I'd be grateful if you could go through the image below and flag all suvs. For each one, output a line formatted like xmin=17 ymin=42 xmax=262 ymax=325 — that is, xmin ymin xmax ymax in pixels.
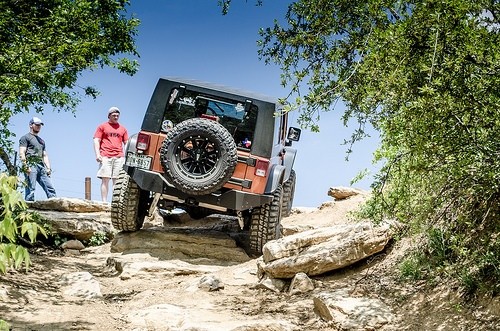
xmin=110 ymin=78 xmax=301 ymax=254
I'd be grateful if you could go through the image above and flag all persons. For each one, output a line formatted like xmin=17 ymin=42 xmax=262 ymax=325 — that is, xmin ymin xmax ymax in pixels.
xmin=94 ymin=106 xmax=128 ymax=203
xmin=18 ymin=117 xmax=58 ymax=202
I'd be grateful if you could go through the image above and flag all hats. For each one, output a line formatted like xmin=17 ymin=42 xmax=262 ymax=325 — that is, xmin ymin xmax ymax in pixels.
xmin=29 ymin=117 xmax=44 ymax=126
xmin=108 ymin=107 xmax=120 ymax=119
